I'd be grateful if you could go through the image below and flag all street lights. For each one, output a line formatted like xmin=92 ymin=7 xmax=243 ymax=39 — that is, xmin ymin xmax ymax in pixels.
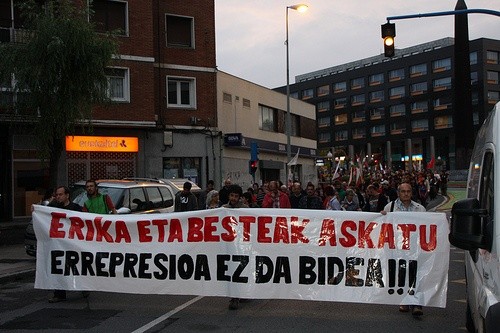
xmin=281 ymin=3 xmax=311 ymax=186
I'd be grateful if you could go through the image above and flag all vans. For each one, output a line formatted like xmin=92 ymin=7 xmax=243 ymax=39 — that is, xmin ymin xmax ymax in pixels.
xmin=449 ymin=100 xmax=500 ymax=333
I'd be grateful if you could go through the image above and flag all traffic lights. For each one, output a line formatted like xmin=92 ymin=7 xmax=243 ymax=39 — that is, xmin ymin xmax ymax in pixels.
xmin=248 ymin=160 xmax=257 ymax=175
xmin=381 ymin=23 xmax=396 ymax=58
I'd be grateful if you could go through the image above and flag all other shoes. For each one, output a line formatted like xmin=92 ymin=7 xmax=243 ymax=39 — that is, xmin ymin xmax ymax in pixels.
xmin=410 ymin=306 xmax=423 ymax=315
xmin=228 ymin=299 xmax=240 ymax=310
xmin=47 ymin=295 xmax=66 ymax=303
xmin=399 ymin=305 xmax=409 ymax=312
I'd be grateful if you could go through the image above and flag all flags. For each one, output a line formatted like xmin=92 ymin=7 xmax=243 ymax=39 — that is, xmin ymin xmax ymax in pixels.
xmin=287 ymin=151 xmax=298 ymax=165
xmin=332 ymin=158 xmax=385 ymax=186
xmin=427 ymin=157 xmax=435 ymax=170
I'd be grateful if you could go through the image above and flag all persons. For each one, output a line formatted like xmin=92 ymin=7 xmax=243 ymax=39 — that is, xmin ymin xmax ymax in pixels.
xmin=206 ymin=190 xmax=219 ymax=209
xmin=262 ymin=180 xmax=323 ymax=210
xmin=83 ymin=180 xmax=117 ymax=214
xmin=30 ymin=186 xmax=90 ymax=303
xmin=222 ymin=184 xmax=251 ymax=310
xmin=389 ymin=160 xmax=448 ymax=208
xmin=320 ymin=182 xmax=389 ymax=213
xmin=241 ymin=183 xmax=265 ymax=208
xmin=197 ymin=180 xmax=215 ymax=210
xmin=219 ymin=178 xmax=232 ymax=204
xmin=319 ymin=161 xmax=390 ymax=193
xmin=175 ymin=181 xmax=198 ymax=213
xmin=380 ymin=182 xmax=426 ymax=316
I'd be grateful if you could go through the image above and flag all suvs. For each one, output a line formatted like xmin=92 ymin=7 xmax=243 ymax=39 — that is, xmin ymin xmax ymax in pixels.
xmin=25 ymin=176 xmax=177 ymax=259
